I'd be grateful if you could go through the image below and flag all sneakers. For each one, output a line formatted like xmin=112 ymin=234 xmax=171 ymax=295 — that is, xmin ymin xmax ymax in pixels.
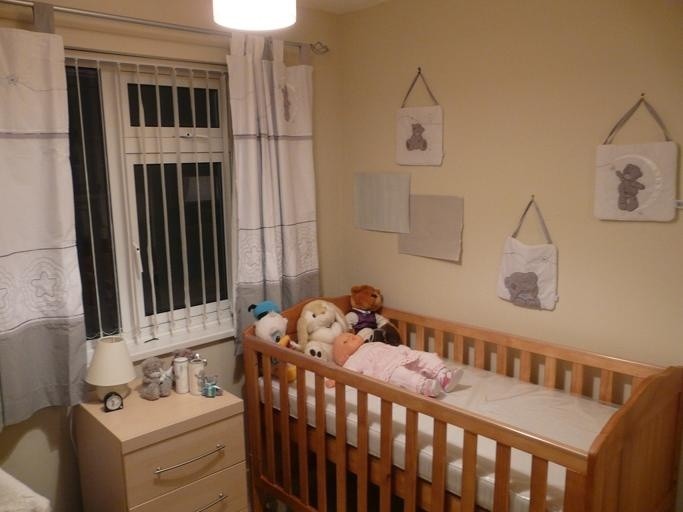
xmin=439 ymin=369 xmax=464 ymax=392
xmin=424 ymin=379 xmax=440 ymax=398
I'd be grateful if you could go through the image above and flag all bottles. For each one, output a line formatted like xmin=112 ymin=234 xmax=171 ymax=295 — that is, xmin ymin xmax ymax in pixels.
xmin=175 ymin=357 xmax=204 ymax=396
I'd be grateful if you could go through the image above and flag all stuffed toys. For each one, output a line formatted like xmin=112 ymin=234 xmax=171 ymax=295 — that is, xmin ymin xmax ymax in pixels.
xmin=141 ymin=357 xmax=174 ymax=400
xmin=246 ymin=301 xmax=299 ymax=383
xmin=344 ymin=284 xmax=399 ymax=346
xmin=296 ymin=299 xmax=350 ymax=362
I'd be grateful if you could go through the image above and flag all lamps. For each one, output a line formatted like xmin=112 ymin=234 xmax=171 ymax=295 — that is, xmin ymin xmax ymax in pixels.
xmin=83 ymin=335 xmax=136 ymax=401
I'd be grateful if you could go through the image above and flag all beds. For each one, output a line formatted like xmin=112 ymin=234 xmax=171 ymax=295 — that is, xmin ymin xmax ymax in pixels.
xmin=248 ymin=294 xmax=681 ymax=511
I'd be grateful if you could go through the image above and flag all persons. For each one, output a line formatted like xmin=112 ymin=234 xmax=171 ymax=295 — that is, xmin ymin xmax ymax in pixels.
xmin=324 ymin=331 xmax=465 ymax=397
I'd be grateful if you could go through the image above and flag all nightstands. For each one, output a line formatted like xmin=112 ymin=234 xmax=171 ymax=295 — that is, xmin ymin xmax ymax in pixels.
xmin=71 ymin=386 xmax=249 ymax=512
